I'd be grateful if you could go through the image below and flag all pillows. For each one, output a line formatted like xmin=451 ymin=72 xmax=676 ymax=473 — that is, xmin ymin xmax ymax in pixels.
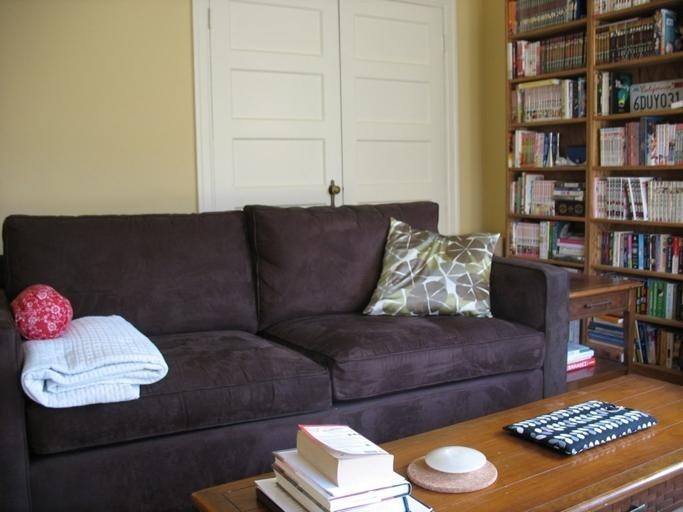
xmin=363 ymin=215 xmax=500 ymax=318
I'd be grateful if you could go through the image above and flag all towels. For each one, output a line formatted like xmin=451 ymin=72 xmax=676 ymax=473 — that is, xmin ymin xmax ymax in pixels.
xmin=11 ymin=284 xmax=168 ymax=409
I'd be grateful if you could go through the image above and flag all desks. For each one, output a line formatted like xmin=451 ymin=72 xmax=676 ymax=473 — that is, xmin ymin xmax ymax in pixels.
xmin=191 ymin=370 xmax=683 ymax=512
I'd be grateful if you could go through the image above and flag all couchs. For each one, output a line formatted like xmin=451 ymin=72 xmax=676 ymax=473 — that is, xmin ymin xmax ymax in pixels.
xmin=0 ymin=202 xmax=569 ymax=512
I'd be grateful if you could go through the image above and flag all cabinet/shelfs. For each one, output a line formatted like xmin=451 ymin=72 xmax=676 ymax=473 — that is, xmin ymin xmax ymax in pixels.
xmin=503 ymin=0 xmax=683 ymax=382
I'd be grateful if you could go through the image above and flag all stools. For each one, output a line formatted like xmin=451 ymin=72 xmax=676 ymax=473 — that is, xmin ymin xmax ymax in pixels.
xmin=567 ymin=276 xmax=644 ymax=377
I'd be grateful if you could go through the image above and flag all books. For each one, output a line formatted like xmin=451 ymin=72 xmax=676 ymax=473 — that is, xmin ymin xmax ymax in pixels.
xmin=503 ymin=1 xmax=586 ymax=124
xmin=592 ymin=117 xmax=682 ymax=224
xmin=508 ymin=128 xmax=586 ymax=272
xmin=593 ymin=1 xmax=683 ymax=115
xmin=567 ymin=230 xmax=682 ymax=372
xmin=251 ymin=422 xmax=432 ymax=512
